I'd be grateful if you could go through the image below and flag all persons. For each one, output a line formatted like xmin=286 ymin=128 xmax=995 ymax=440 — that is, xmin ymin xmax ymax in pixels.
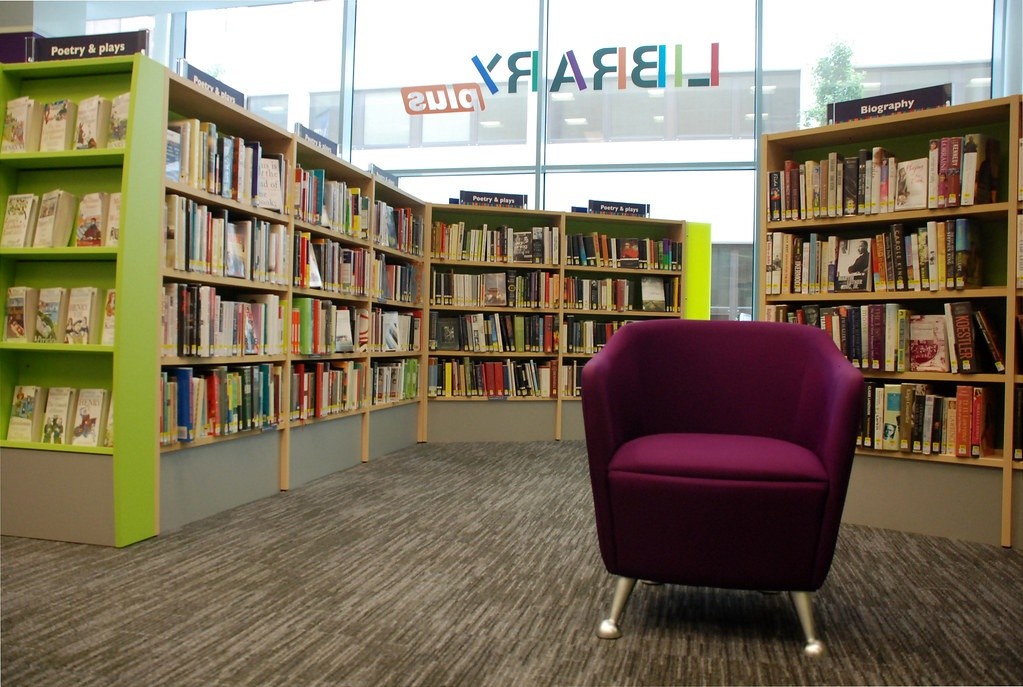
xmin=949 ymin=401 xmax=956 ymax=410
xmin=805 ymin=307 xmax=818 ymax=327
xmin=848 ymin=240 xmax=869 ymax=289
xmin=883 ymin=423 xmax=895 ymax=440
xmin=965 ymin=136 xmax=977 ymax=152
xmin=839 ymin=241 xmax=847 ymax=254
xmin=930 ymin=140 xmax=938 ymax=152
xmin=977 ymin=139 xmax=1001 ymax=203
xmin=882 ymin=159 xmax=888 ymax=167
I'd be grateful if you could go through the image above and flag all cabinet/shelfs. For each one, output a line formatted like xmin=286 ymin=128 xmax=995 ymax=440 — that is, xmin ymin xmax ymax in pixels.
xmin=757 ymin=95 xmax=1023 ymax=548
xmin=1 ymin=51 xmax=713 ymax=548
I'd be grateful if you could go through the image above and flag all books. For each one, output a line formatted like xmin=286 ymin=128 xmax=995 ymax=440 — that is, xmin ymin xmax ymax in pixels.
xmin=0 ymin=93 xmax=684 ymax=447
xmin=765 ymin=135 xmax=1022 ymax=461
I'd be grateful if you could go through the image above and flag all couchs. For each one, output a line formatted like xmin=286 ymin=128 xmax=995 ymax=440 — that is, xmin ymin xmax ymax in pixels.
xmin=581 ymin=321 xmax=865 ymax=661
